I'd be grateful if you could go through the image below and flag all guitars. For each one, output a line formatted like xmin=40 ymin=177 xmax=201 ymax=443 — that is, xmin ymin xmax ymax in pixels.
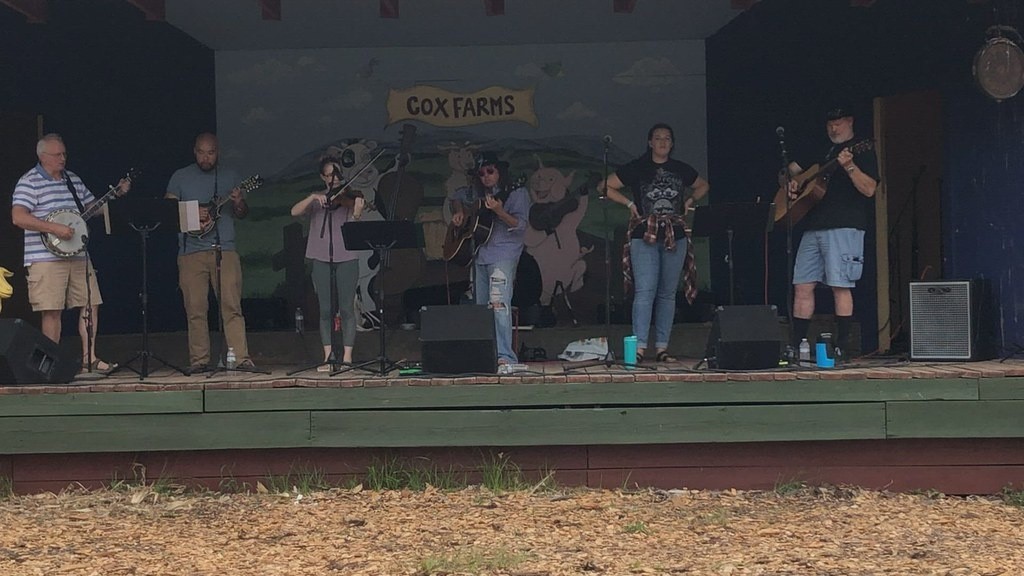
xmin=39 ymin=166 xmax=144 ymax=257
xmin=771 ymin=137 xmax=878 ymax=233
xmin=185 ymin=172 xmax=265 ymax=236
xmin=443 ymin=172 xmax=527 ymax=269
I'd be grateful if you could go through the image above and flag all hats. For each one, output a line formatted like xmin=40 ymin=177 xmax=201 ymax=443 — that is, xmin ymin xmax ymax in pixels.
xmin=468 ymin=150 xmax=510 ymax=176
xmin=824 ymin=103 xmax=852 ymax=121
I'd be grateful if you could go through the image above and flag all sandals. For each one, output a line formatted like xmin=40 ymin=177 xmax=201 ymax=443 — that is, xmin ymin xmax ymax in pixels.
xmin=657 ymin=350 xmax=676 ymax=362
xmin=637 ymin=353 xmax=645 ymax=363
xmin=80 ymin=357 xmax=119 ymax=373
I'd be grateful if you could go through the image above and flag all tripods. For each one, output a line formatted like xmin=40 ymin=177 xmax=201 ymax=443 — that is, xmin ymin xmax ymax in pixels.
xmin=106 ymin=223 xmax=189 ymax=378
xmin=195 ymin=200 xmax=272 ymax=378
xmin=330 ymin=219 xmax=425 ymax=377
xmin=565 ymin=152 xmax=655 ymax=371
xmin=878 ymin=173 xmax=925 ymax=356
xmin=284 ymin=205 xmax=380 ymax=375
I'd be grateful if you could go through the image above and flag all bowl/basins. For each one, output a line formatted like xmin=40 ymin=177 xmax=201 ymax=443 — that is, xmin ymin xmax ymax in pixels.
xmin=402 ymin=323 xmax=415 ymax=330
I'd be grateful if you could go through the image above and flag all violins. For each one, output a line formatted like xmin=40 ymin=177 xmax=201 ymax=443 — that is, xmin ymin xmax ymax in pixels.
xmin=342 ymin=183 xmax=376 ymax=212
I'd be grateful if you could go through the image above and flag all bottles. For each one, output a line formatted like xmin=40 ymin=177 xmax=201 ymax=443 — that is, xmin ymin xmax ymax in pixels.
xmin=799 ymin=338 xmax=810 ymax=368
xmin=227 ymin=347 xmax=236 ymax=377
xmin=295 ymin=308 xmax=305 ymax=333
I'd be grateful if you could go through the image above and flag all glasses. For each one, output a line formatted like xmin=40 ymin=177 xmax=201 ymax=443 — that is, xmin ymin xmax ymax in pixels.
xmin=476 ymin=169 xmax=496 ymax=178
xmin=43 ymin=152 xmax=66 ymax=159
xmin=323 ymin=173 xmax=333 ymax=178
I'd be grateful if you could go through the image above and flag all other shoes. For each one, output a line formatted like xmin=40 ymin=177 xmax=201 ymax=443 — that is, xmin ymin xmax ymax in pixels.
xmin=498 ymin=357 xmax=508 ymax=365
xmin=238 ymin=363 xmax=252 ymax=369
xmin=830 ymin=346 xmax=846 ymax=362
xmin=316 ymin=362 xmax=337 ymax=372
xmin=340 ymin=363 xmax=351 ymax=371
xmin=191 ymin=366 xmax=207 ymax=373
xmin=783 ymin=345 xmax=800 ymax=361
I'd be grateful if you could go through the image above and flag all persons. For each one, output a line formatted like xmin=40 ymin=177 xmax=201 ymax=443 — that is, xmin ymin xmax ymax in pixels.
xmin=290 ymin=156 xmax=365 ymax=372
xmin=452 ymin=151 xmax=531 ymax=366
xmin=12 ymin=133 xmax=130 ymax=375
xmin=596 ymin=124 xmax=709 ymax=363
xmin=159 ymin=133 xmax=257 ymax=372
xmin=785 ymin=105 xmax=882 ymax=365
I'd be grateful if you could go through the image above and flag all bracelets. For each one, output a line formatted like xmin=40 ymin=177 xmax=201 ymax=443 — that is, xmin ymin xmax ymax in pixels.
xmin=690 ymin=195 xmax=698 ymax=204
xmin=627 ymin=201 xmax=634 ymax=209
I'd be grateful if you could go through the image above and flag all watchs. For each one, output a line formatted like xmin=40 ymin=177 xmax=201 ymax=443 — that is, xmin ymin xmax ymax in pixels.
xmin=847 ymin=164 xmax=857 ymax=175
xmin=352 ymin=212 xmax=361 ymax=219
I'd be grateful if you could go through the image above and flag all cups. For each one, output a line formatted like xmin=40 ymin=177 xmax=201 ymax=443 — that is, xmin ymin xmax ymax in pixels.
xmin=624 ymin=336 xmax=638 ymax=369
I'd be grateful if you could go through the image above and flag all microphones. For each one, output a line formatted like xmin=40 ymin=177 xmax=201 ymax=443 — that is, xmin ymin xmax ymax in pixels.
xmin=601 ymin=135 xmax=613 ymax=144
xmin=333 ymin=165 xmax=346 ymax=185
xmin=775 ymin=126 xmax=785 ymax=143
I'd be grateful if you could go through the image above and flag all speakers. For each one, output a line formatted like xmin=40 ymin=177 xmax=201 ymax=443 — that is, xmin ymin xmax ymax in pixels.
xmin=419 ymin=304 xmax=498 ymax=375
xmin=0 ymin=319 xmax=58 ymax=385
xmin=705 ymin=304 xmax=781 ymax=370
xmin=909 ymin=280 xmax=973 ymax=360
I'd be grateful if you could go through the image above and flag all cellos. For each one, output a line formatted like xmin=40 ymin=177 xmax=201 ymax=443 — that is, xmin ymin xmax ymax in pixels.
xmin=370 ymin=123 xmax=425 ymax=296
